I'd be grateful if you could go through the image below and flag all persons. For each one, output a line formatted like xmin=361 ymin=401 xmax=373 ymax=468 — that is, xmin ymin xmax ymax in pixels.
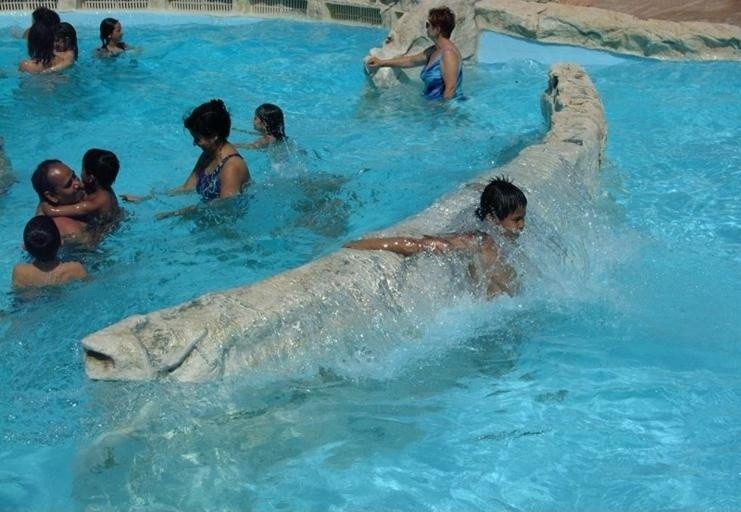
xmin=233 ymin=104 xmax=288 ymax=150
xmin=0 ymin=138 xmax=127 ymax=289
xmin=18 ymin=7 xmax=78 ymax=75
xmin=96 ymin=18 xmax=144 ymax=57
xmin=341 ymin=174 xmax=528 ymax=301
xmin=367 ymin=7 xmax=467 ymax=102
xmin=120 ymin=99 xmax=250 ymax=222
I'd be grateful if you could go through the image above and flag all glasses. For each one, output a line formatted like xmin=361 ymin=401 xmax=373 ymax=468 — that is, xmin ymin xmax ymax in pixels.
xmin=426 ymin=22 xmax=431 ymax=28
